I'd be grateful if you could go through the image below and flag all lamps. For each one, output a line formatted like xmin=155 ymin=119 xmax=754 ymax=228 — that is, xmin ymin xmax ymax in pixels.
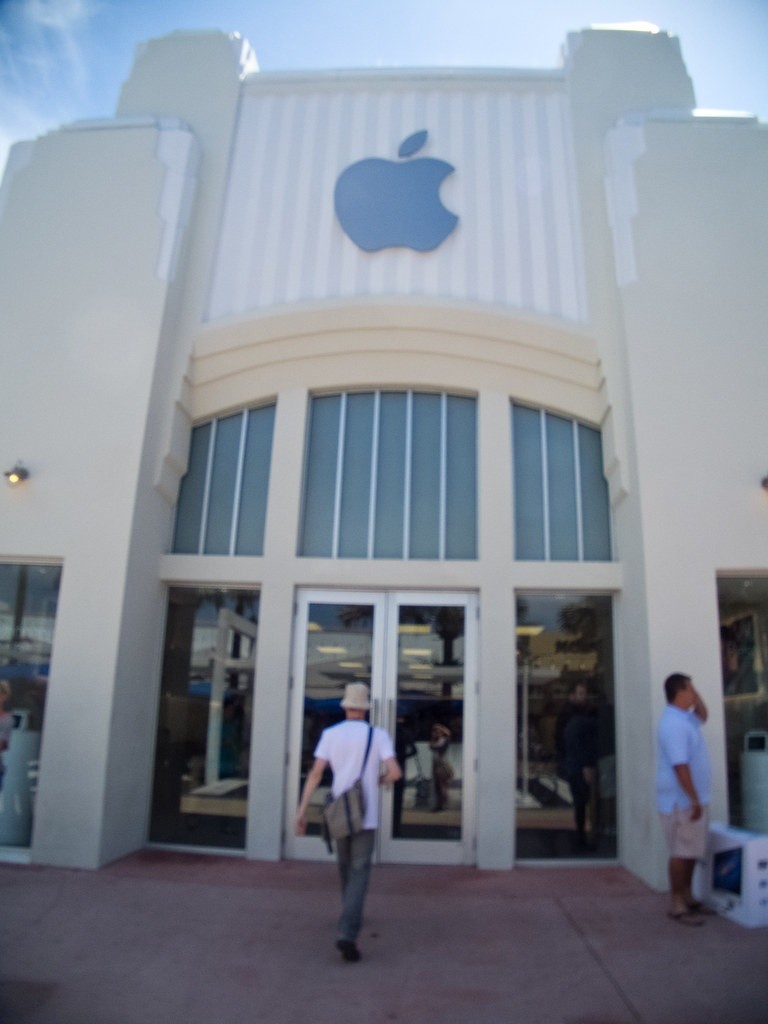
xmin=3 ymin=464 xmax=28 ymax=484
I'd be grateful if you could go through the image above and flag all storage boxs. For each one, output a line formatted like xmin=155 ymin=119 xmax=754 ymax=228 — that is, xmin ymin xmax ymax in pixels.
xmin=692 ymin=821 xmax=768 ymax=929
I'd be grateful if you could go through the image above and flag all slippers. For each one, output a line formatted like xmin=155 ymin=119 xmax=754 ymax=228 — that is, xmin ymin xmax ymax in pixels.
xmin=667 ymin=909 xmax=704 ymax=926
xmin=689 ymin=901 xmax=715 ymax=915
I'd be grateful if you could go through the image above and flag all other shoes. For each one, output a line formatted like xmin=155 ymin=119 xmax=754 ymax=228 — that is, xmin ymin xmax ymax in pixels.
xmin=336 ymin=940 xmax=361 ymax=962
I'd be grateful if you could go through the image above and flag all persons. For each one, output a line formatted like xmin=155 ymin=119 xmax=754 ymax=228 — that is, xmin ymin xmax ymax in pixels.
xmin=296 ymin=682 xmax=403 ymax=964
xmin=654 ymin=674 xmax=716 ymax=925
xmin=555 ymin=684 xmax=606 ymax=840
xmin=0 ymin=679 xmax=15 ymax=793
xmin=431 ymin=724 xmax=454 ymax=813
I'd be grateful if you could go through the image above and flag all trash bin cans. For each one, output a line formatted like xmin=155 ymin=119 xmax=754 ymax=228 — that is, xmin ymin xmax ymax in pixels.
xmin=0 ymin=709 xmax=39 ymax=845
xmin=741 ymin=729 xmax=768 ymax=834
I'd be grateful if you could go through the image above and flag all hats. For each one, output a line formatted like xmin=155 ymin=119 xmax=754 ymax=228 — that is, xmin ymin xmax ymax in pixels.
xmin=340 ymin=683 xmax=371 ymax=710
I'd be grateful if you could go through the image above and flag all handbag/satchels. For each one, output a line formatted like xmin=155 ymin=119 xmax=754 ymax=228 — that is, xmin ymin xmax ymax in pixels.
xmin=323 ymin=782 xmax=367 ymax=840
